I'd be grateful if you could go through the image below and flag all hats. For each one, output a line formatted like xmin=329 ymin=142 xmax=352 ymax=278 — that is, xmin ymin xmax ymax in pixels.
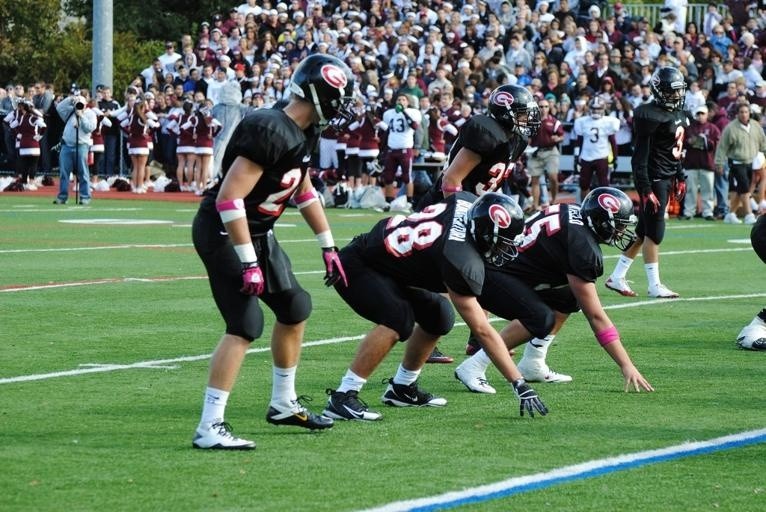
xmin=693 ymin=104 xmax=708 ymax=115
xmin=96 ymin=84 xmax=105 ymax=91
xmin=754 ymin=79 xmax=766 ymax=87
xmin=201 ymin=3 xmax=684 ymax=106
xmin=165 ymin=41 xmax=174 ymax=48
xmin=70 ymin=82 xmax=81 ymax=92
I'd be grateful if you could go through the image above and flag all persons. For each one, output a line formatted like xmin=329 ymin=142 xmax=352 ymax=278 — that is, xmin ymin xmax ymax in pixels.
xmin=736 ymin=214 xmax=766 ymax=350
xmin=0 ymin=81 xmax=222 ymax=195
xmin=324 ymin=192 xmax=548 ymax=420
xmin=454 ymin=188 xmax=654 ymax=394
xmin=456 ymin=0 xmax=766 ymax=191
xmin=528 ymin=99 xmax=563 ymax=214
xmin=136 ymin=0 xmax=455 ymax=209
xmin=605 ymin=69 xmax=687 ymax=297
xmin=54 ymin=94 xmax=97 ymax=205
xmin=574 ymin=96 xmax=619 ymax=203
xmin=684 ymin=100 xmax=766 ymax=225
xmin=417 ymin=85 xmax=540 ymax=361
xmin=382 ymin=95 xmax=421 ymax=211
xmin=194 ymin=55 xmax=355 ymax=456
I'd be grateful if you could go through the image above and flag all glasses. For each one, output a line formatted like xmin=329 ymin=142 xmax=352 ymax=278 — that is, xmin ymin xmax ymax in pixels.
xmin=538 ymin=104 xmax=549 ymax=109
xmin=5 ymin=85 xmax=43 ymax=92
xmin=661 ymin=87 xmax=682 ymax=95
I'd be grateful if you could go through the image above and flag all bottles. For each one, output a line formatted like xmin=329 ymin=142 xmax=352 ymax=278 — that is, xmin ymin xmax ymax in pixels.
xmin=395 ymin=103 xmax=402 ymax=114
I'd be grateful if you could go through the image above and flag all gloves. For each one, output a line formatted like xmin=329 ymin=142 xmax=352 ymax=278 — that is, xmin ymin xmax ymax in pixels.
xmin=673 ymin=177 xmax=687 ymax=203
xmin=238 ymin=260 xmax=266 ymax=297
xmin=319 ymin=245 xmax=350 ymax=289
xmin=511 ymin=377 xmax=550 ymax=419
xmin=641 ymin=190 xmax=662 ymax=216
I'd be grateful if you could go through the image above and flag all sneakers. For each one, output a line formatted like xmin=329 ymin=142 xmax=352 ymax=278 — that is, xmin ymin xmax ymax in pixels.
xmin=646 ymin=282 xmax=681 ymax=298
xmin=603 ymin=275 xmax=639 ymax=298
xmin=131 ymin=179 xmax=155 ymax=194
xmin=722 ymin=212 xmax=742 ymax=225
xmin=23 ymin=179 xmax=45 ymax=191
xmin=744 ymin=212 xmax=757 ymax=225
xmin=53 ymin=199 xmax=68 ymax=205
xmin=379 ymin=376 xmax=449 ymax=409
xmin=463 ymin=341 xmax=517 ymax=359
xmin=262 ymin=395 xmax=335 ymax=431
xmin=425 ymin=344 xmax=454 ymax=364
xmin=452 ymin=366 xmax=498 ymax=397
xmin=513 ymin=363 xmax=574 ymax=385
xmin=733 ymin=322 xmax=766 ymax=353
xmin=179 ymin=180 xmax=209 ymax=196
xmin=321 ymin=388 xmax=384 ymax=422
xmin=78 ymin=198 xmax=92 ymax=206
xmin=677 ymin=213 xmax=695 ymax=223
xmin=191 ymin=420 xmax=258 ymax=452
xmin=704 ymin=214 xmax=718 ymax=222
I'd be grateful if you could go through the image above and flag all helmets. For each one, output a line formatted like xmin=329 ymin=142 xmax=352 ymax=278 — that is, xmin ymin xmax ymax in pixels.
xmin=487 ymin=83 xmax=543 ymax=138
xmin=649 ymin=66 xmax=688 ymax=111
xmin=287 ymin=52 xmax=357 ymax=131
xmin=587 ymin=96 xmax=606 ymax=120
xmin=577 ymin=185 xmax=639 ymax=254
xmin=464 ymin=190 xmax=526 ymax=267
